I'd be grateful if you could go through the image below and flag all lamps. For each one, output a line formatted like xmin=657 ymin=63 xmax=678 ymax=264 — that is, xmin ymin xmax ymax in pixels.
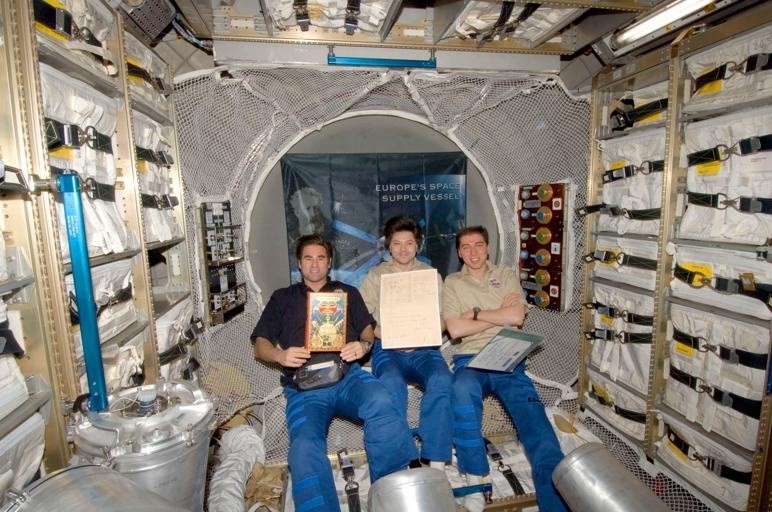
xmin=609 ymin=0 xmax=718 ymax=52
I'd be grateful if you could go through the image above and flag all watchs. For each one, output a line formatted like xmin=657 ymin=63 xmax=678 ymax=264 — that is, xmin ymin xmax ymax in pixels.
xmin=471 ymin=306 xmax=482 ymax=321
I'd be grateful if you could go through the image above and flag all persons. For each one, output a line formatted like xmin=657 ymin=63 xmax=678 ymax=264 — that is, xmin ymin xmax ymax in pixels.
xmin=249 ymin=232 xmax=416 ymax=510
xmin=286 ymin=186 xmax=359 ymax=270
xmin=356 ymin=217 xmax=454 ymax=473
xmin=443 ymin=224 xmax=571 ymax=512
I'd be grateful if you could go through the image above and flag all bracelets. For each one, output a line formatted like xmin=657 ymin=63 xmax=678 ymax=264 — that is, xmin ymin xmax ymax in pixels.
xmin=360 ymin=338 xmax=372 ymax=353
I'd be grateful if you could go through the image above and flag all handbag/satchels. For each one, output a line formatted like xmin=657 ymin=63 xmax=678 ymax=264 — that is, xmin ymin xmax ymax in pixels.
xmin=295 ymin=353 xmax=343 ymax=390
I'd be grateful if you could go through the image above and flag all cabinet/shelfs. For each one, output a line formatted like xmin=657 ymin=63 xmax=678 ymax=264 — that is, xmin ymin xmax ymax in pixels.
xmin=1 ymin=0 xmax=206 ymax=481
xmin=579 ymin=17 xmax=772 ymax=509
xmin=212 ymin=0 xmax=655 ymax=59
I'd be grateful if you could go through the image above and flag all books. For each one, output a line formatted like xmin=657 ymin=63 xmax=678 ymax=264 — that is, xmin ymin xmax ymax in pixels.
xmin=302 ymin=289 xmax=348 ymax=354
